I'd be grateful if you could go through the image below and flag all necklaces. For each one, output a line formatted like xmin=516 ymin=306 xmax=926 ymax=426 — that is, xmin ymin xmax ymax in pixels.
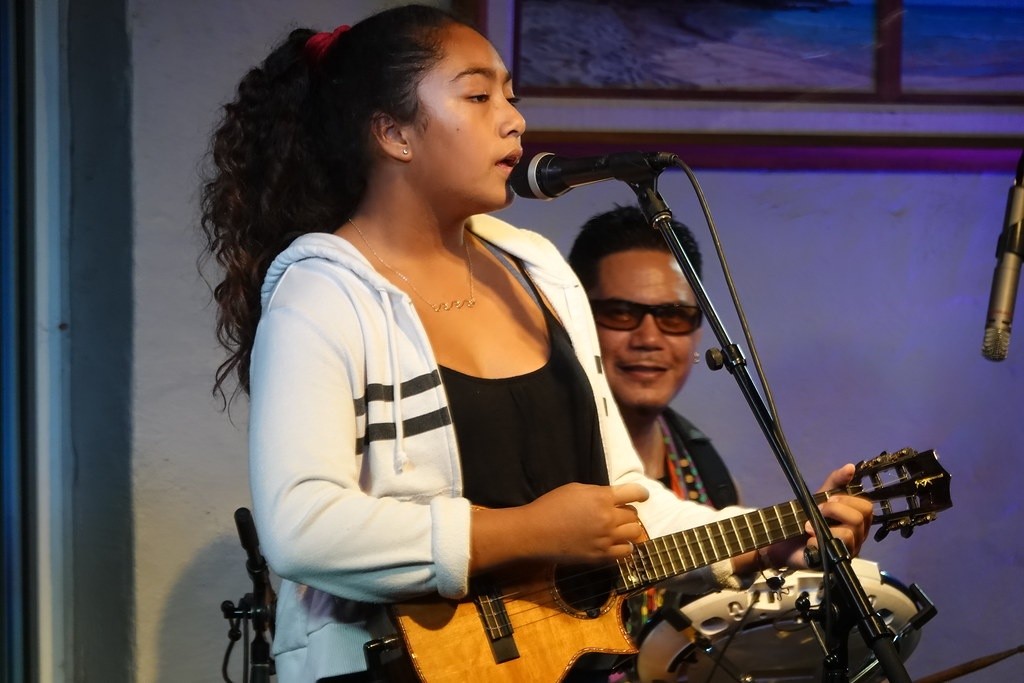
xmin=346 ymin=213 xmax=477 ymax=314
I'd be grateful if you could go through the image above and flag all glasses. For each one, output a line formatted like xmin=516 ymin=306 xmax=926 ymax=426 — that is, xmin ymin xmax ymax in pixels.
xmin=587 ymin=299 xmax=701 ymax=336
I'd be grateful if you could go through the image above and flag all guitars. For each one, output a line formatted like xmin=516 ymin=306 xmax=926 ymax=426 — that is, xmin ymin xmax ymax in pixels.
xmin=387 ymin=445 xmax=952 ymax=683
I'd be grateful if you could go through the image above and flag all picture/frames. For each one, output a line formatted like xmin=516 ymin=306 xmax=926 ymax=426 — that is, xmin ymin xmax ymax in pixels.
xmin=475 ymin=0 xmax=1024 ymax=175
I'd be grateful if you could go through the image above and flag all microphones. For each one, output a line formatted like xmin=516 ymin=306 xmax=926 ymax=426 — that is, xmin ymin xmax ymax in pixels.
xmin=510 ymin=151 xmax=674 ymax=201
xmin=982 ymin=185 xmax=1024 ymax=362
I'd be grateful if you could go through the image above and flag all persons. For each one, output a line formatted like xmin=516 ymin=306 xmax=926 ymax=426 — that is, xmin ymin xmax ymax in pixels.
xmin=565 ymin=202 xmax=741 ymax=638
xmin=199 ymin=6 xmax=873 ymax=682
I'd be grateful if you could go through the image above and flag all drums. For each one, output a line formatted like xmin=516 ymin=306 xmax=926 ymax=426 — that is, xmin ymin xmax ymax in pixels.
xmin=617 ymin=534 xmax=923 ymax=682
